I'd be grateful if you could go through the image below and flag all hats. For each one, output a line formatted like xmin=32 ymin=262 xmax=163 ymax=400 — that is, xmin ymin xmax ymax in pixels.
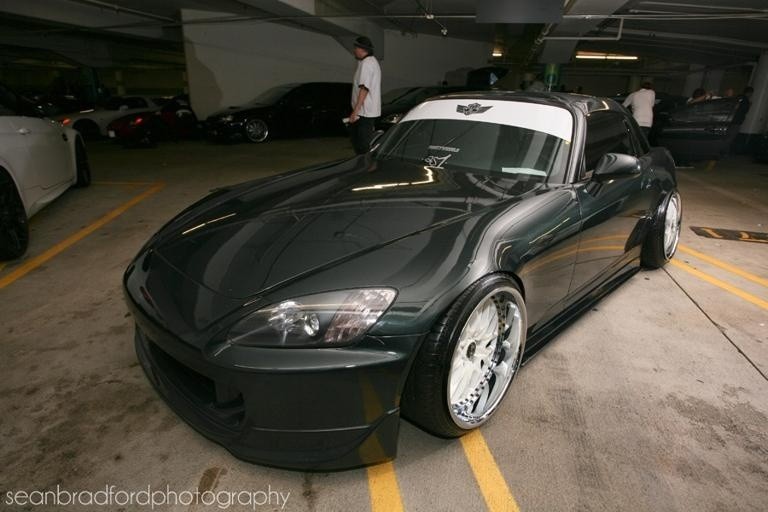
xmin=352 ymin=36 xmax=375 ymax=49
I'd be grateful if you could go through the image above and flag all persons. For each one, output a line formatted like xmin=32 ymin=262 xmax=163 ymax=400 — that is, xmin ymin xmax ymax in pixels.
xmin=347 ymin=36 xmax=384 ymax=153
xmin=491 ymin=64 xmax=751 ymax=127
xmin=623 ymin=82 xmax=654 ymax=147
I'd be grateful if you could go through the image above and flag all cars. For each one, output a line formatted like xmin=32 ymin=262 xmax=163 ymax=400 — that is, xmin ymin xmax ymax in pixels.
xmin=204 ymin=83 xmax=353 ymax=142
xmin=610 ymin=92 xmax=744 ymax=167
xmin=374 ymin=68 xmax=513 ymax=137
xmin=50 ymin=94 xmax=162 ymax=138
xmin=0 ymin=80 xmax=92 ymax=259
xmin=106 ymin=94 xmax=191 ymax=146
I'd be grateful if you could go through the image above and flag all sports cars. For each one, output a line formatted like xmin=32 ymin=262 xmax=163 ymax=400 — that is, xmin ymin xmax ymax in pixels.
xmin=122 ymin=90 xmax=683 ymax=473
